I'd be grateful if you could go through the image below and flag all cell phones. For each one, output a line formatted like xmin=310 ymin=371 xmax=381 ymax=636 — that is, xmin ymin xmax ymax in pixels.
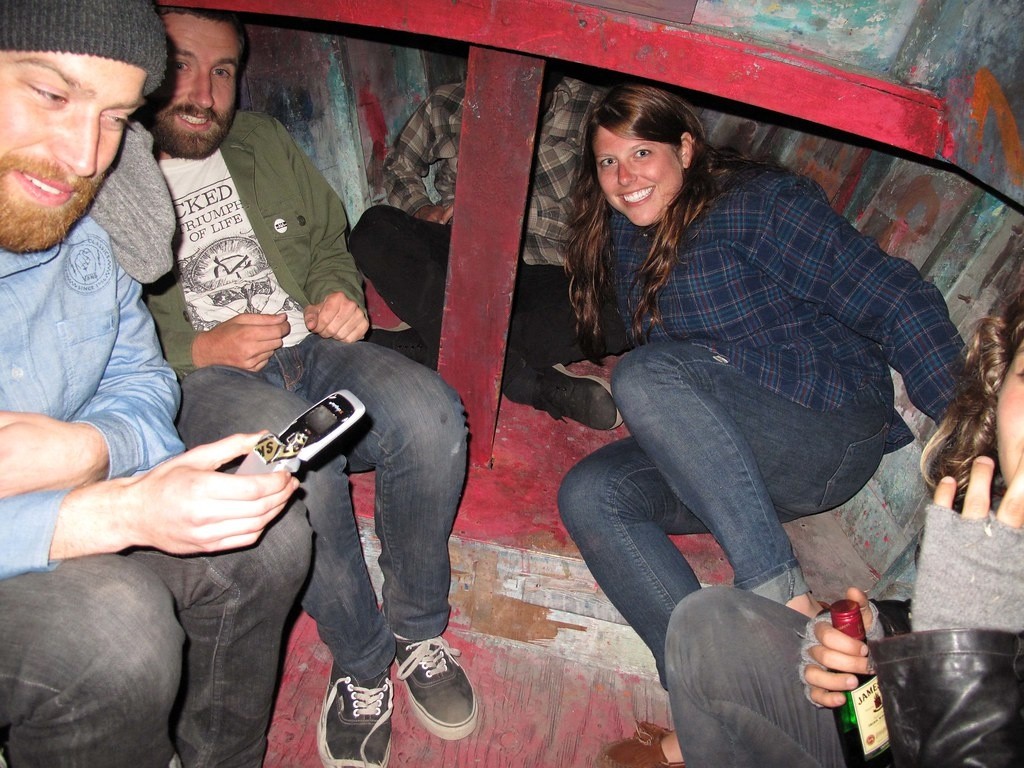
xmin=234 ymin=389 xmax=366 ymax=475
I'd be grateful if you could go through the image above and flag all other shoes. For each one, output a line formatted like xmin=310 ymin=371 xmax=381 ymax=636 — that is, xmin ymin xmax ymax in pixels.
xmin=594 ymin=720 xmax=687 ymax=768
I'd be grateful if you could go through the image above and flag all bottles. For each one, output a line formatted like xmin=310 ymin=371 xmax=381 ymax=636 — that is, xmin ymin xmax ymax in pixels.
xmin=826 ymin=599 xmax=892 ymax=768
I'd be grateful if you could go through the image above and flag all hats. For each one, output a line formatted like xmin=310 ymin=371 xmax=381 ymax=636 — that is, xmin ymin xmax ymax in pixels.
xmin=0 ymin=0 xmax=166 ymax=96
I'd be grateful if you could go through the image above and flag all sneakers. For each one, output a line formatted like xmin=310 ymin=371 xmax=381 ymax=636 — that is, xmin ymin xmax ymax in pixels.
xmin=316 ymin=660 xmax=393 ymax=768
xmin=548 ymin=363 xmax=623 ymax=430
xmin=381 ymin=605 xmax=478 ymax=740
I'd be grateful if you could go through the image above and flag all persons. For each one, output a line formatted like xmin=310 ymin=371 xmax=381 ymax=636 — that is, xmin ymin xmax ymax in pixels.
xmin=1 ymin=0 xmax=315 ymax=768
xmin=349 ymin=75 xmax=624 ymax=431
xmin=666 ymin=291 xmax=1023 ymax=767
xmin=143 ymin=10 xmax=477 ymax=768
xmin=557 ymin=86 xmax=967 ymax=767
